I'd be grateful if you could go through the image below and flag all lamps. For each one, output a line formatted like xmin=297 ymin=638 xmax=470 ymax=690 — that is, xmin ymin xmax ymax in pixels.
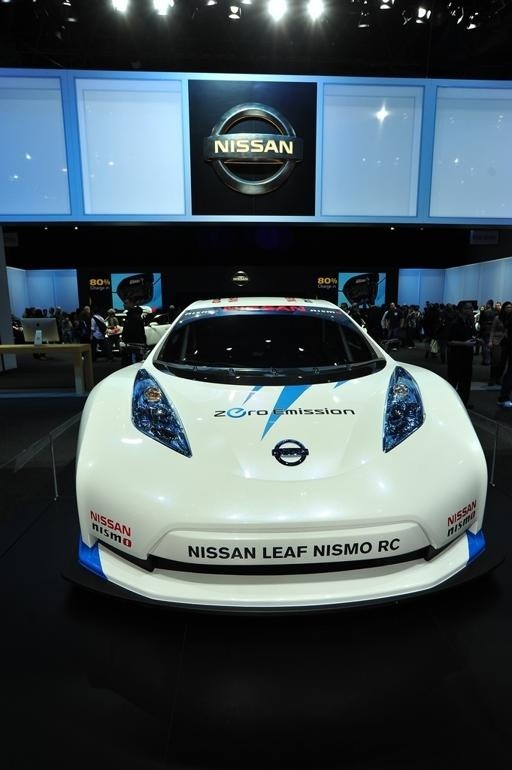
xmin=227 ymin=0 xmax=248 ymax=21
xmin=347 ymin=0 xmax=482 ymax=31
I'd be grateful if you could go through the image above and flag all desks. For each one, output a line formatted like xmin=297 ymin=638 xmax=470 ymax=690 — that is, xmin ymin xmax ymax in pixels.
xmin=0 ymin=340 xmax=94 ymax=400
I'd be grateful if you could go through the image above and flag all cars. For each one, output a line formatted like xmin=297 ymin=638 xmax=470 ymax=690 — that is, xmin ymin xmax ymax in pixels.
xmin=90 ymin=305 xmax=176 ymax=357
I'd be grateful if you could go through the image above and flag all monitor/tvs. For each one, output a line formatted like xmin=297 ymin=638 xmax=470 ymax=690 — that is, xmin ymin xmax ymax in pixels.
xmin=21 ymin=317 xmax=60 ymax=345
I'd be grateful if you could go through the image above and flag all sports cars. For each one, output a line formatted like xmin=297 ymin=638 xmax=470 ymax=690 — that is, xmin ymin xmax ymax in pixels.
xmin=70 ymin=294 xmax=490 ymax=623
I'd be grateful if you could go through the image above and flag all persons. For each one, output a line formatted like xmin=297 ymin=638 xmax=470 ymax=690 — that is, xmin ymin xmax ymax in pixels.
xmin=342 ymin=299 xmax=512 ymax=408
xmin=23 ymin=298 xmax=175 ymax=364
